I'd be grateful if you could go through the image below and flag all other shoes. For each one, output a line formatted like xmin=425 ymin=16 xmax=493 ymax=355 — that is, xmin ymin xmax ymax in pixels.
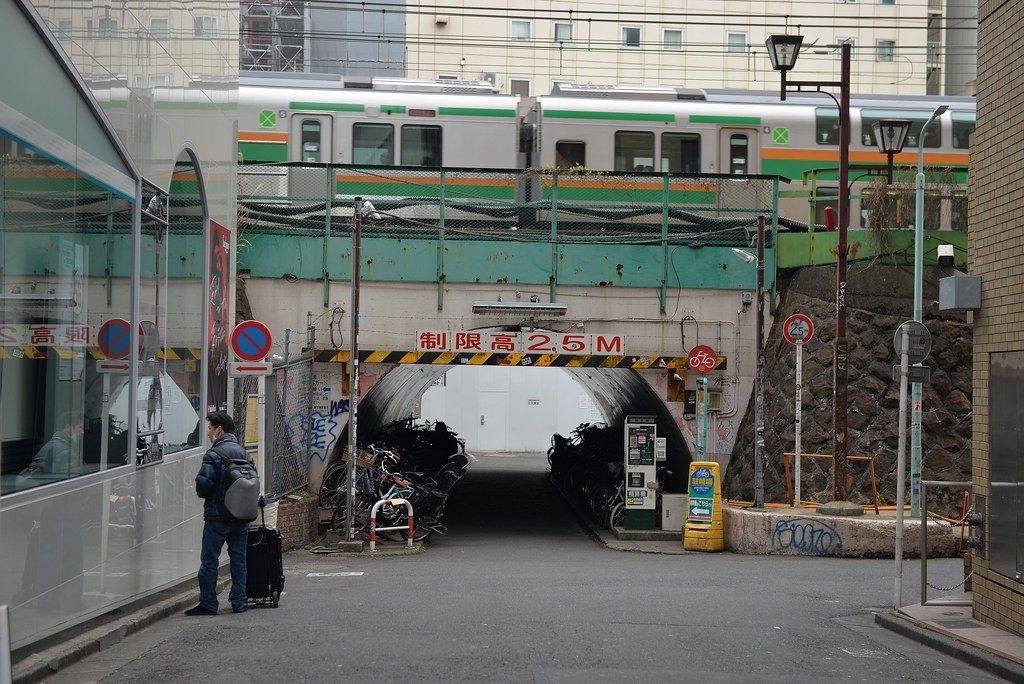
xmin=233 ymin=604 xmax=246 ymax=613
xmin=185 ymin=603 xmax=218 ymax=616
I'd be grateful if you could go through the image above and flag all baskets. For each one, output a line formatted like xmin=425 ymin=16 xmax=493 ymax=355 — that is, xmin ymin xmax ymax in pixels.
xmin=341 ymin=445 xmax=377 ymax=467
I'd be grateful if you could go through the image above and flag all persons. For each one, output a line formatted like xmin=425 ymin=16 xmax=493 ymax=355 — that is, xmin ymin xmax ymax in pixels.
xmin=185 ymin=412 xmax=255 ymax=614
xmin=28 ymin=410 xmax=91 ymax=615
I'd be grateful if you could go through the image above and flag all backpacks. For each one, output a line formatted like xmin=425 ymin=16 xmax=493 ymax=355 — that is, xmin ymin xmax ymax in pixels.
xmin=15 ymin=444 xmax=71 ymax=491
xmin=208 ymin=447 xmax=260 ymax=520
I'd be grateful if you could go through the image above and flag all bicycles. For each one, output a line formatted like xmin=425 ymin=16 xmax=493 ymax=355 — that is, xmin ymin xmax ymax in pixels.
xmin=319 ymin=411 xmax=472 ymax=543
xmin=547 ymin=421 xmax=674 ymax=532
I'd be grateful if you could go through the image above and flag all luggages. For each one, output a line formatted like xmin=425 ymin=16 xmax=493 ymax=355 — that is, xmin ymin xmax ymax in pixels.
xmin=242 ymin=500 xmax=285 ymax=608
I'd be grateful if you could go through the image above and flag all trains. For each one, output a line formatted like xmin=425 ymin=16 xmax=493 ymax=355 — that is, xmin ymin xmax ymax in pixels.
xmin=0 ymin=68 xmax=978 ymax=232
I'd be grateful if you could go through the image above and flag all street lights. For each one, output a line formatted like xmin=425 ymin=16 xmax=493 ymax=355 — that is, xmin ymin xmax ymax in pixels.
xmin=765 ymin=34 xmax=914 ymax=501
xmin=907 ymin=104 xmax=952 ymax=519
